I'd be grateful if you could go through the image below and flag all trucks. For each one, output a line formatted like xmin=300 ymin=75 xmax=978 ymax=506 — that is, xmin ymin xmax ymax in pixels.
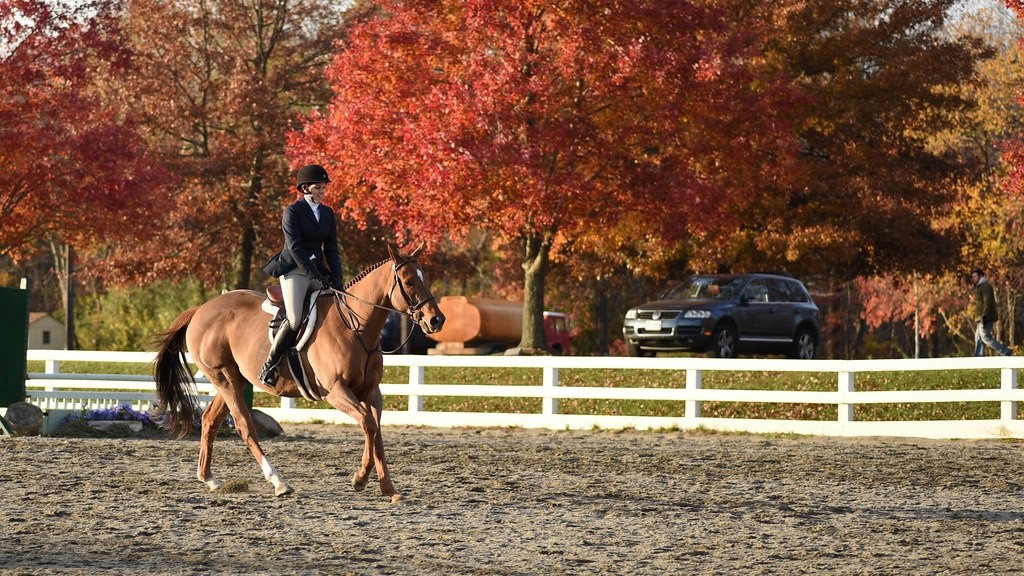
xmin=422 ymin=294 xmax=572 ymax=356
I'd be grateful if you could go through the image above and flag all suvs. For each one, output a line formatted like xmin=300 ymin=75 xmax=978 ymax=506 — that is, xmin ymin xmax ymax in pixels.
xmin=622 ymin=273 xmax=822 ymax=358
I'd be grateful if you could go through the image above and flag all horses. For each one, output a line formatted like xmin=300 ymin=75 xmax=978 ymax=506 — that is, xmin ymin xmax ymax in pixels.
xmin=137 ymin=241 xmax=447 ymax=504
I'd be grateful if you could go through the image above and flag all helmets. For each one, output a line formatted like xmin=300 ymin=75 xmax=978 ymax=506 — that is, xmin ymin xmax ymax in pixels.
xmin=296 ymin=164 xmax=332 ymax=195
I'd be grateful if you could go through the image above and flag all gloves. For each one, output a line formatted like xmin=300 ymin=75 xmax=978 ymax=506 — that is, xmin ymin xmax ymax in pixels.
xmin=981 ymin=316 xmax=987 ymax=329
xmin=319 ymin=270 xmax=343 ymax=292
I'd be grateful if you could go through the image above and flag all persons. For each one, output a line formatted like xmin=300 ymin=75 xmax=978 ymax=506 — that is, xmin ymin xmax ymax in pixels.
xmin=257 ymin=164 xmax=345 ymax=389
xmin=970 ymin=266 xmax=1013 ymax=356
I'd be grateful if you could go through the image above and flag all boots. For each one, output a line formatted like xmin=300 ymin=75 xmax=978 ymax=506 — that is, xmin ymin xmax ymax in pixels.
xmin=258 ymin=318 xmax=297 ymax=386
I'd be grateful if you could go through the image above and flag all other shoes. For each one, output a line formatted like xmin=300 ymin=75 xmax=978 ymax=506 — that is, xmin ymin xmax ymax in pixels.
xmin=1005 ymin=349 xmax=1013 ymax=356
